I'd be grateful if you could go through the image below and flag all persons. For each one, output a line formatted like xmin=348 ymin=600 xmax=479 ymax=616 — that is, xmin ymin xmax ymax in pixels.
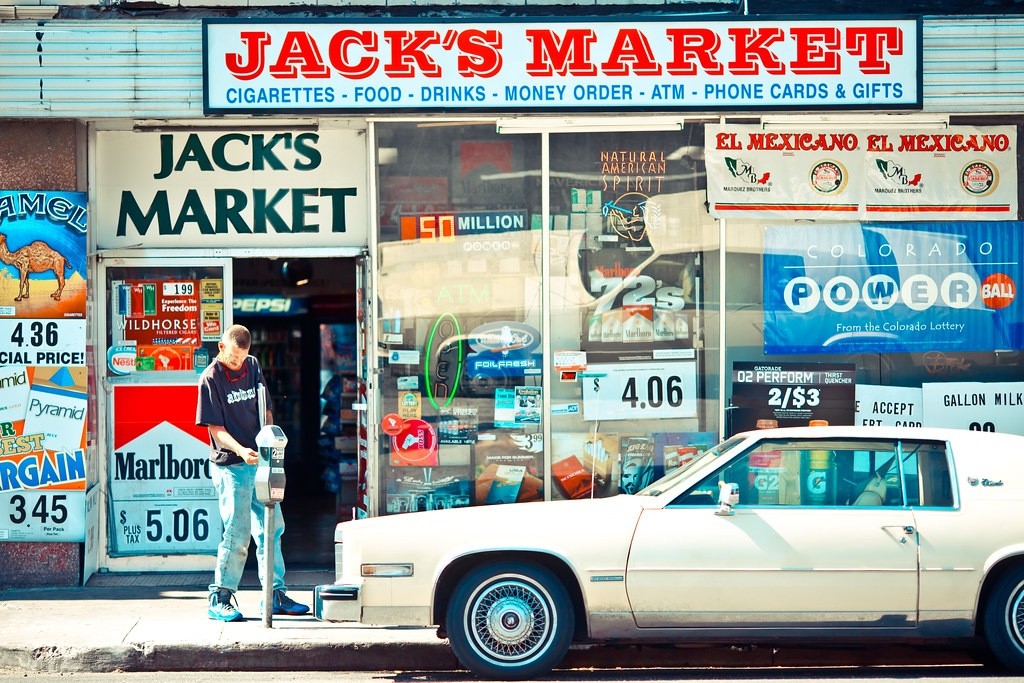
xmin=195 ymin=324 xmax=310 ymax=623
xmin=618 ymin=463 xmax=641 ymax=495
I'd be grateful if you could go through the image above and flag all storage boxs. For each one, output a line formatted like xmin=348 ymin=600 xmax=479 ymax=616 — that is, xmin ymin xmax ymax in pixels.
xmin=339 ymin=459 xmax=357 ymax=474
xmin=335 ymin=437 xmax=356 ymax=450
xmin=340 ymin=409 xmax=357 ymax=419
xmin=342 ymin=482 xmax=357 ymax=503
xmin=342 ymin=377 xmax=357 ymax=393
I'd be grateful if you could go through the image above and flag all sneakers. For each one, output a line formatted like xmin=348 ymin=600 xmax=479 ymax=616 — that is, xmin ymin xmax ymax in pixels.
xmin=207 ymin=587 xmax=243 ymax=622
xmin=260 ymin=588 xmax=310 ymax=614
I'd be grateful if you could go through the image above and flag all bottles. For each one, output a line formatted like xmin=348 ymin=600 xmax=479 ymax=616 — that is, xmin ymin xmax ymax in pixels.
xmin=247 ymin=317 xmax=290 ymax=424
xmin=748 ymin=420 xmax=780 ymax=505
xmin=799 ymin=420 xmax=837 ymax=506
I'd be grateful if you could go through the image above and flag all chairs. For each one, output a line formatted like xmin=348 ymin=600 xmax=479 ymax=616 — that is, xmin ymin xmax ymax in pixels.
xmin=855 ymin=473 xmax=889 ymax=508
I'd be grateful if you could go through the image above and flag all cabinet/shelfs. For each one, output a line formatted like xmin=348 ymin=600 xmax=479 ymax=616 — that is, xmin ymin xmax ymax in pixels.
xmin=335 ymin=370 xmax=360 ymax=521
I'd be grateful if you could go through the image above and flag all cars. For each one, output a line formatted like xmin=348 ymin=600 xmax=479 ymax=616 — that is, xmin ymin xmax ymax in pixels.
xmin=312 ymin=425 xmax=1023 ymax=675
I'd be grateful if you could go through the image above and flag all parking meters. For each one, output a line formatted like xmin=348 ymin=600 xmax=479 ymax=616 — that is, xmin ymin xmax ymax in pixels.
xmin=253 ymin=423 xmax=288 ymax=627
